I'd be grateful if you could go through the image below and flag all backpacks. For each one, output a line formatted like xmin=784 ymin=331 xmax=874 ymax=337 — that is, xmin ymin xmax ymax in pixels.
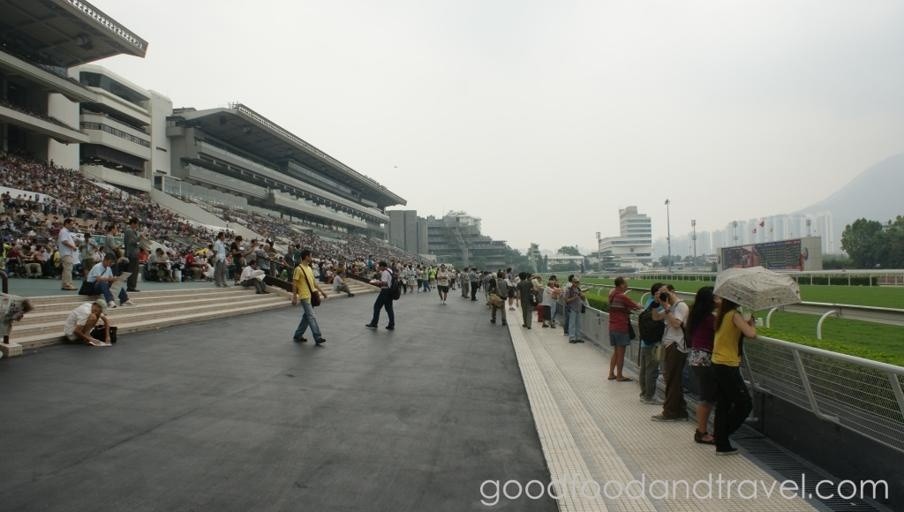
xmin=528 ymin=289 xmax=538 ymax=305
xmin=390 ymin=273 xmax=401 ymax=300
xmin=489 ymin=293 xmax=504 ymax=307
xmin=638 ymin=301 xmax=665 ymax=346
xmin=494 ymin=287 xmax=509 ymax=301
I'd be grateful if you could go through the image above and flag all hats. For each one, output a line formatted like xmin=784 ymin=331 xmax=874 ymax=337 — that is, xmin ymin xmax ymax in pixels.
xmin=571 ymin=277 xmax=580 ymax=283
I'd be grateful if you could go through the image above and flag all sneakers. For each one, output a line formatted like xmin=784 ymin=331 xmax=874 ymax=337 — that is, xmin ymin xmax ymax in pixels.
xmin=106 ymin=299 xmax=117 ymax=308
xmin=294 ymin=336 xmax=307 ymax=343
xmin=638 ymin=394 xmax=688 ymax=421
xmin=715 ymin=445 xmax=740 ymax=456
xmin=126 ymin=287 xmax=140 ymax=292
xmin=489 ymin=319 xmax=496 ymax=324
xmin=256 ymin=290 xmax=270 ymax=295
xmin=62 ymin=282 xmax=77 ymax=291
xmin=316 ymin=337 xmax=326 ymax=345
xmin=441 ymin=300 xmax=447 ymax=305
xmin=119 ymin=299 xmax=138 ymax=307
xmin=217 ymin=283 xmax=230 ymax=287
xmin=462 ymin=294 xmax=480 ymax=302
xmin=501 ymin=319 xmax=508 ymax=325
xmin=521 ymin=319 xmax=584 ymax=344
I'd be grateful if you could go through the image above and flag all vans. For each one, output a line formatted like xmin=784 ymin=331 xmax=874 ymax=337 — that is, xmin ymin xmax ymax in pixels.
xmin=705 ymin=256 xmax=719 ymax=264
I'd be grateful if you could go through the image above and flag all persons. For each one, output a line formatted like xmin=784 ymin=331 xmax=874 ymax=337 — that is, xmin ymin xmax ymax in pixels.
xmin=393 ymin=258 xmax=592 ymax=344
xmin=84 ymin=251 xmax=135 ymax=308
xmin=364 ymin=261 xmax=400 ymax=331
xmin=288 ymin=248 xmax=329 ymax=346
xmin=605 ymin=275 xmax=758 ymax=458
xmin=60 ymin=298 xmax=111 ymax=350
xmin=271 ymin=215 xmax=429 ymax=298
xmin=0 ymin=150 xmax=271 ymax=294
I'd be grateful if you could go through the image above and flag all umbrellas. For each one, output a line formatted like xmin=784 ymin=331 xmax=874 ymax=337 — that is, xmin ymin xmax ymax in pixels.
xmin=712 ymin=265 xmax=802 ymax=315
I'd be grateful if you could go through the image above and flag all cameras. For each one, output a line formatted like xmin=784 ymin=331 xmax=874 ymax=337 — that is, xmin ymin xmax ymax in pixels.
xmin=659 ymin=292 xmax=670 ymax=302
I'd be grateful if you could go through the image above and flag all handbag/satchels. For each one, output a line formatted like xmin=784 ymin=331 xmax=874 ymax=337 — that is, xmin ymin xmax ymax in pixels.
xmin=90 ymin=325 xmax=117 ymax=343
xmin=311 ymin=291 xmax=322 ymax=307
xmin=78 ymin=280 xmax=98 ymax=295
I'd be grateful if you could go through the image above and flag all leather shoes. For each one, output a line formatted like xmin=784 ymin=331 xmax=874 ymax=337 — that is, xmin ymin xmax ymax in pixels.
xmin=386 ymin=326 xmax=394 ymax=330
xmin=366 ymin=324 xmax=378 ymax=329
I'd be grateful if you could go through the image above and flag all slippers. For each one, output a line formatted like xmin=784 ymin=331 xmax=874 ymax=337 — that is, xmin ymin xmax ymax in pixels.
xmin=616 ymin=375 xmax=634 ymax=382
xmin=608 ymin=376 xmax=616 ymax=380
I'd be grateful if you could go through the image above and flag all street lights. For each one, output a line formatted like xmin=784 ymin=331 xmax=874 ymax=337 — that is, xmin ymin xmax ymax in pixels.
xmin=732 ymin=220 xmax=738 ymax=245
xmin=595 ymin=231 xmax=601 ymax=272
xmin=664 ymin=197 xmax=672 ymax=273
xmin=690 ymin=219 xmax=697 ymax=256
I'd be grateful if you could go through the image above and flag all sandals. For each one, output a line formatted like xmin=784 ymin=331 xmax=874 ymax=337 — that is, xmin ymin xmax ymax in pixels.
xmin=693 ymin=429 xmax=717 ymax=445
